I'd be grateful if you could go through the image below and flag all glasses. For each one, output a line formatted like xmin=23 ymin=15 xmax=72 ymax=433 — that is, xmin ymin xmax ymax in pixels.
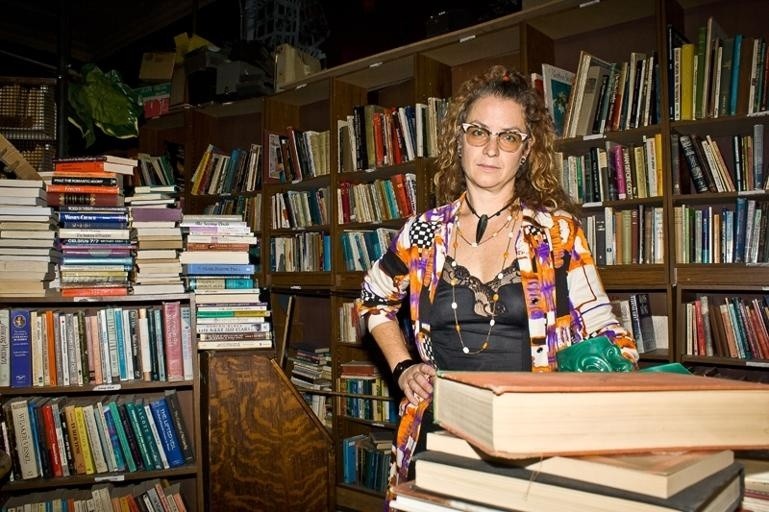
xmin=461 ymin=122 xmax=528 ymax=153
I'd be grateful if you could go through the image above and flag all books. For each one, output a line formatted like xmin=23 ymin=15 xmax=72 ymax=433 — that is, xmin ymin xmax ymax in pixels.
xmin=665 ymin=17 xmax=769 ymax=263
xmin=679 ymin=294 xmax=768 ymax=381
xmin=389 ymin=370 xmax=767 ymax=511
xmin=337 ymin=97 xmax=444 ymax=272
xmin=1 ymin=143 xmax=274 ymax=510
xmin=609 ymin=293 xmax=656 ymax=353
xmin=529 ymin=47 xmax=664 ymax=267
xmin=264 ymin=128 xmax=331 ymax=271
xmin=340 ymin=298 xmax=367 ymax=346
xmin=287 ymin=344 xmax=334 ymax=429
xmin=335 ymin=359 xmax=398 ymax=497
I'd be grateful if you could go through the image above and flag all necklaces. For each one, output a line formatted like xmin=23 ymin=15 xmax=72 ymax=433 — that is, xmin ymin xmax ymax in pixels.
xmin=451 ymin=201 xmax=520 ymax=355
xmin=464 ymin=193 xmax=518 ymax=244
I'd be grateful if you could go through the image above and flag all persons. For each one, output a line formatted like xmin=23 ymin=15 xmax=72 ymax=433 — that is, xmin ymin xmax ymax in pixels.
xmin=353 ymin=64 xmax=641 ymax=511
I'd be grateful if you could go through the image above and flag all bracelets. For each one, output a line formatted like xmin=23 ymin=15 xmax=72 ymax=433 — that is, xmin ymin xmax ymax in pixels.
xmin=391 ymin=359 xmax=418 ymax=380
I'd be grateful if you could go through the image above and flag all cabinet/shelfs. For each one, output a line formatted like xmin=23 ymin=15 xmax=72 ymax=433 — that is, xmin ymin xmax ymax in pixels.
xmin=141 ymin=0 xmax=769 ymax=511
xmin=0 ymin=289 xmax=203 ymax=512
